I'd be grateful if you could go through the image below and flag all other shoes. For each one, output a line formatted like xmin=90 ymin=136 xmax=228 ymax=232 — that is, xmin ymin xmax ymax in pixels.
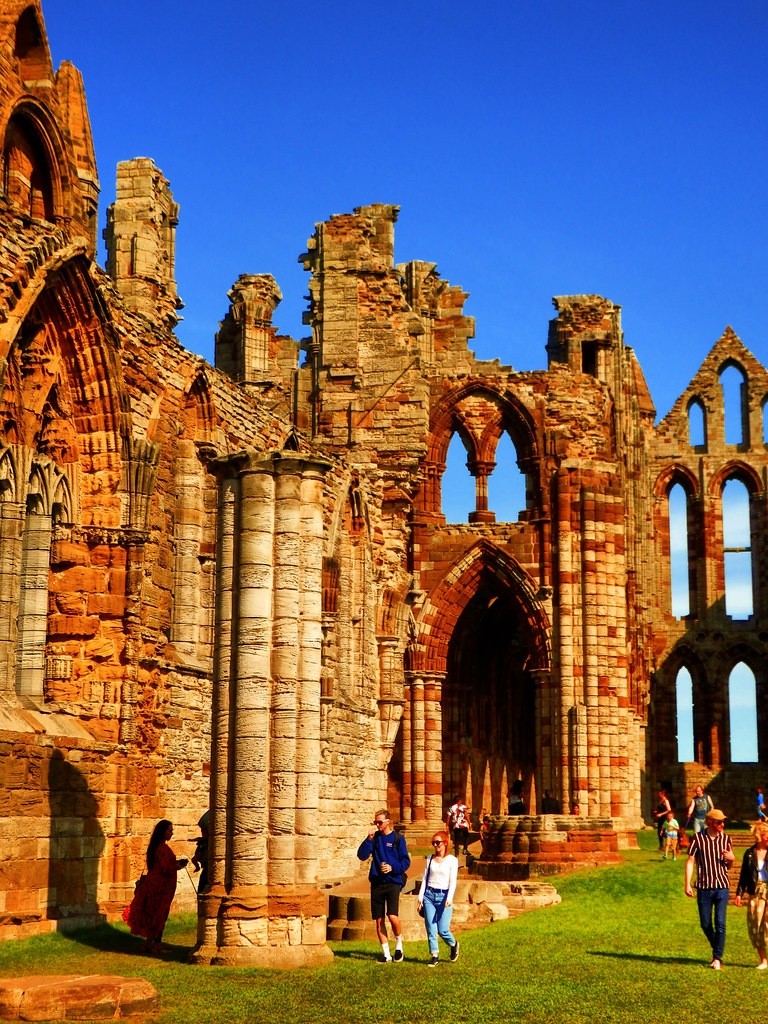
xmin=455 ymin=854 xmax=459 ymax=858
xmin=139 ymin=943 xmax=174 ymax=955
xmin=662 ymin=855 xmax=667 ymax=859
xmin=710 ymin=958 xmax=721 ymax=970
xmin=755 ymin=961 xmax=768 ymax=969
xmin=463 ymin=849 xmax=468 ymax=855
xmin=671 ymin=857 xmax=676 ymax=861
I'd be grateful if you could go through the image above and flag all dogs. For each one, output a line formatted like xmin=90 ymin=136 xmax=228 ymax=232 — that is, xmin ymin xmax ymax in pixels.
xmin=676 ymin=828 xmax=691 ymax=856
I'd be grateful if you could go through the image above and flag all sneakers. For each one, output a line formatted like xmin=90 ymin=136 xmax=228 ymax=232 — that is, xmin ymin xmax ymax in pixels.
xmin=394 ymin=948 xmax=404 ymax=963
xmin=377 ymin=955 xmax=392 ymax=964
xmin=450 ymin=941 xmax=460 ymax=962
xmin=427 ymin=956 xmax=438 ymax=967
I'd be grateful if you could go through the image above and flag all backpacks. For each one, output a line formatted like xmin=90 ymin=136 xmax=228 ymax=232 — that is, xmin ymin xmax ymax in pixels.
xmin=374 ymin=831 xmax=408 ymax=888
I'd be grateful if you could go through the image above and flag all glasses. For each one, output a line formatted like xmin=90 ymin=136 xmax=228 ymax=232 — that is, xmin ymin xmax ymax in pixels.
xmin=432 ymin=840 xmax=444 ymax=846
xmin=373 ymin=820 xmax=387 ymax=826
xmin=713 ymin=821 xmax=725 ymax=825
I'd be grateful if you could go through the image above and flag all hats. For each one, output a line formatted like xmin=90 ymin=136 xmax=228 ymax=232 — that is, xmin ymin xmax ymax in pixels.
xmin=706 ymin=809 xmax=728 ymax=821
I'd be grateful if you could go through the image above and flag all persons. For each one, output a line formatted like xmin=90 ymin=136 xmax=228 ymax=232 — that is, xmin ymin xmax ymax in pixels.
xmin=687 ymin=786 xmax=714 ymax=834
xmin=659 ymin=811 xmax=679 ymax=861
xmin=734 ymin=821 xmax=768 ymax=969
xmin=653 ymin=789 xmax=671 ymax=851
xmin=417 ymin=831 xmax=459 ymax=968
xmin=506 ymin=780 xmax=523 ymax=815
xmin=542 ymin=789 xmax=559 ymax=814
xmin=446 ymin=797 xmax=471 ymax=858
xmin=755 ymin=786 xmax=768 ymax=823
xmin=133 ymin=820 xmax=189 ymax=954
xmin=573 ymin=805 xmax=579 ymax=815
xmin=357 ymin=811 xmax=411 ymax=963
xmin=685 ymin=809 xmax=736 ymax=969
xmin=479 ymin=816 xmax=491 ymax=856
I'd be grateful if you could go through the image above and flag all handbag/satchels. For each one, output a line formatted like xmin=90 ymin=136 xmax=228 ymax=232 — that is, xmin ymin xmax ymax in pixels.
xmin=134 ymin=874 xmax=147 ymax=894
xmin=760 ymin=805 xmax=766 ymax=809
xmin=419 ymin=901 xmax=425 ymax=918
